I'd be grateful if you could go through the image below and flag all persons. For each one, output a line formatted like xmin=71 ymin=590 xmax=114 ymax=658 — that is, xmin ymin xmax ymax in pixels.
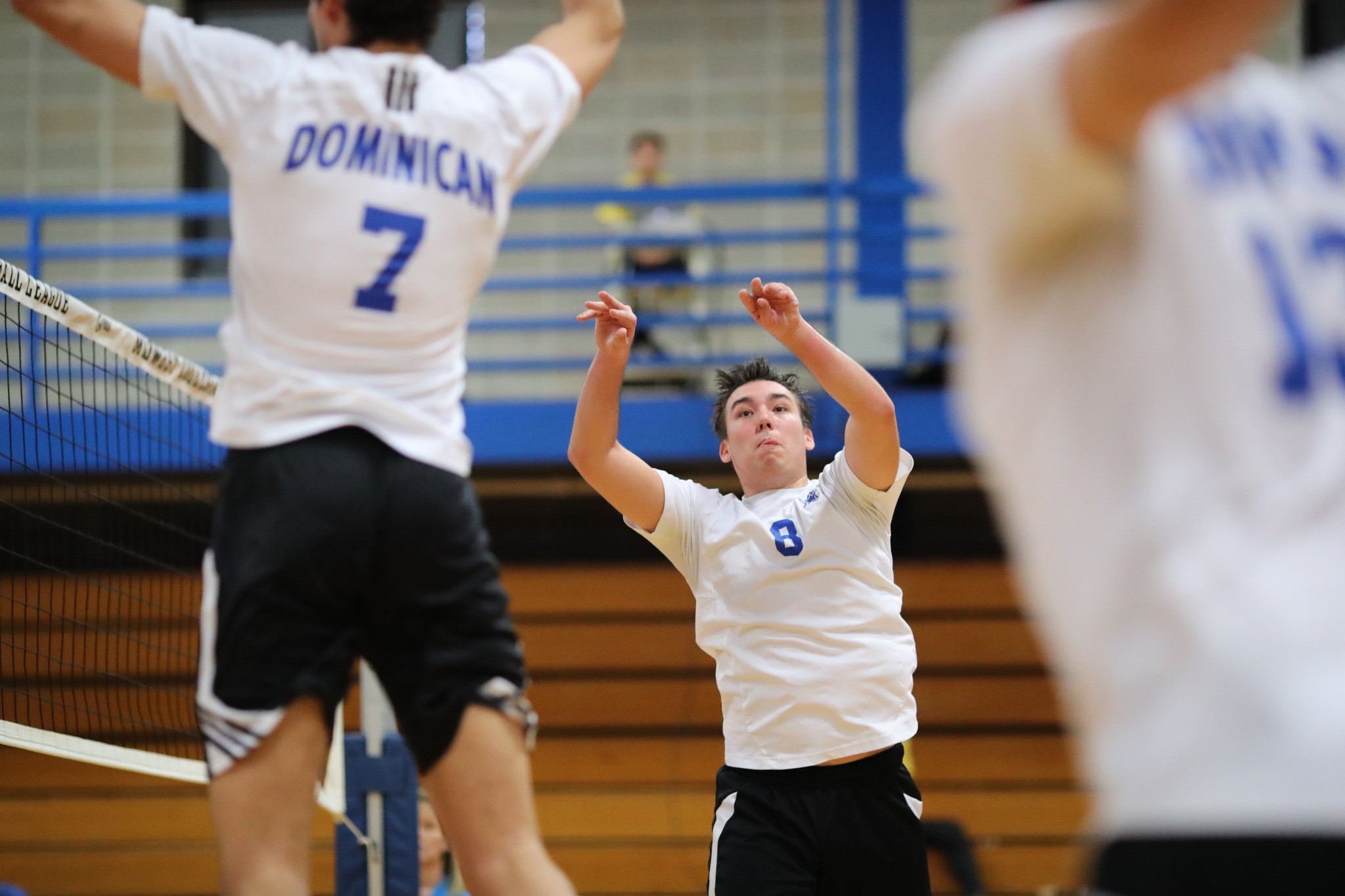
xmin=415 ymin=785 xmax=473 ymax=896
xmin=568 ymin=276 xmax=934 ymax=895
xmin=594 ymin=131 xmax=701 ymax=361
xmin=903 ymin=1 xmax=1345 ymax=896
xmin=0 ymin=0 xmax=624 ymax=896
